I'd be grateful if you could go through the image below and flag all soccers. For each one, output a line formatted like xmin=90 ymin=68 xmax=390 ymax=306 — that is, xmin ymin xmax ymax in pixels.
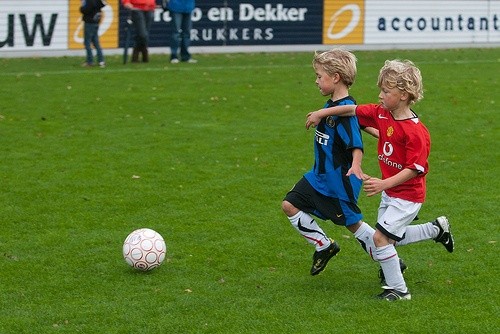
xmin=123 ymin=228 xmax=166 ymax=271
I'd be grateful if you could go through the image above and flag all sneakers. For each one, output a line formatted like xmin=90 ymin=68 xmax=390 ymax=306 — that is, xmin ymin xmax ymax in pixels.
xmin=431 ymin=216 xmax=454 ymax=254
xmin=310 ymin=238 xmax=341 ymax=275
xmin=375 ymin=287 xmax=412 ymax=302
xmin=377 ymin=259 xmax=409 ymax=284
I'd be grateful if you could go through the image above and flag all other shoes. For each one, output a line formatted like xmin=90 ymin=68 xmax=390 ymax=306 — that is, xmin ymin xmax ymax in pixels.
xmin=185 ymin=59 xmax=198 ymax=63
xmin=99 ymin=61 xmax=106 ymax=69
xmin=170 ymin=58 xmax=179 ymax=64
xmin=81 ymin=62 xmax=95 ymax=67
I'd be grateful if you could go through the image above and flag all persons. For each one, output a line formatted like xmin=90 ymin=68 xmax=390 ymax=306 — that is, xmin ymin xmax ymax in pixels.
xmin=80 ymin=0 xmax=105 ymax=68
xmin=167 ymin=0 xmax=198 ymax=64
xmin=306 ymin=60 xmax=455 ymax=302
xmin=282 ymin=48 xmax=408 ymax=284
xmin=121 ymin=0 xmax=156 ymax=63
